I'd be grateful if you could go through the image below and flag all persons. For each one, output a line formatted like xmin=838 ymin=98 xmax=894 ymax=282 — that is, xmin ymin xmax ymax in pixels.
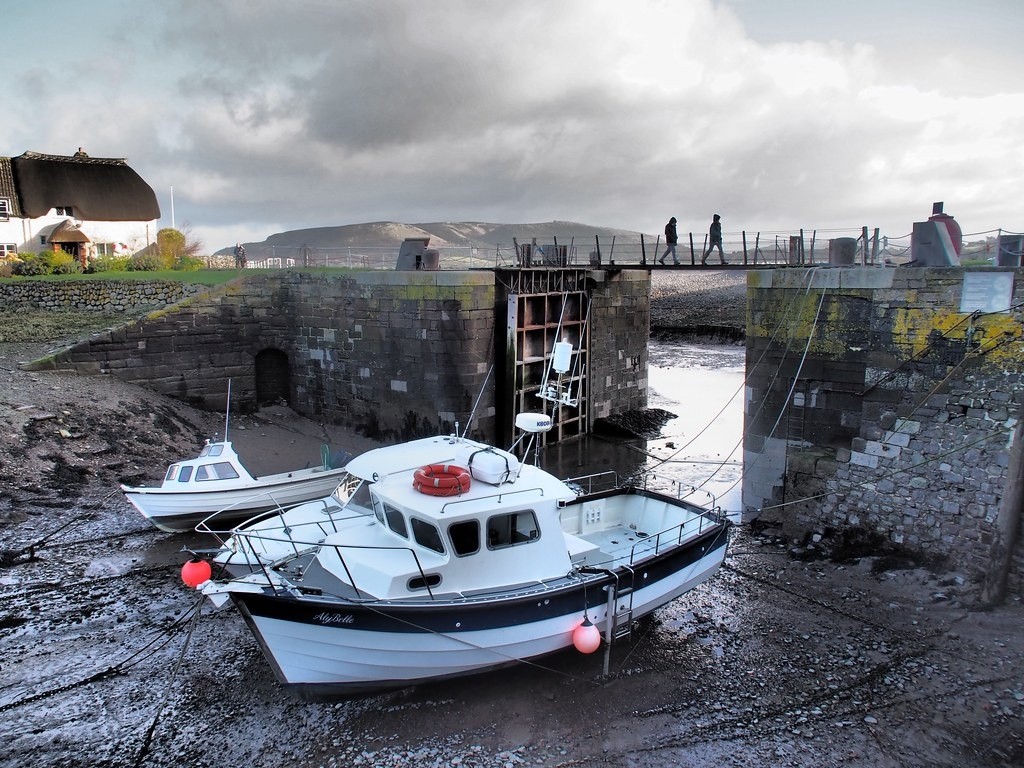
xmin=701 ymin=214 xmax=728 ymax=265
xmin=234 ymin=243 xmax=247 ymax=269
xmin=659 ymin=217 xmax=680 ymax=265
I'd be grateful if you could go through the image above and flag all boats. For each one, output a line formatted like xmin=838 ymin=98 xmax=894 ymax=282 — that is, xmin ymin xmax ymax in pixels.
xmin=118 ymin=376 xmax=346 ymax=536
xmin=180 ymin=289 xmax=733 ymax=704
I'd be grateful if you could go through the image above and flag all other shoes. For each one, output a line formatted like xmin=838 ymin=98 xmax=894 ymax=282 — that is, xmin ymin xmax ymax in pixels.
xmin=721 ymin=260 xmax=729 ymax=265
xmin=658 ymin=259 xmax=665 ymax=265
xmin=674 ymin=261 xmax=680 ymax=265
xmin=701 ymin=260 xmax=707 ymax=265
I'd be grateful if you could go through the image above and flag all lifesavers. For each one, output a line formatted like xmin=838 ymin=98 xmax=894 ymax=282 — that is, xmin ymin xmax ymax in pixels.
xmin=412 ymin=462 xmax=473 ymax=498
